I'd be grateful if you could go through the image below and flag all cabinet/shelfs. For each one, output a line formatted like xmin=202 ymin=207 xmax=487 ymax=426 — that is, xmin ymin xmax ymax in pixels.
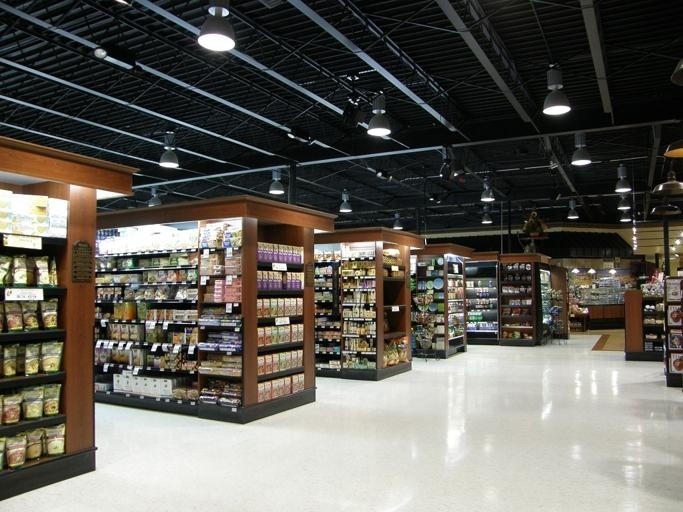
xmin=642 ymin=296 xmax=665 ymax=361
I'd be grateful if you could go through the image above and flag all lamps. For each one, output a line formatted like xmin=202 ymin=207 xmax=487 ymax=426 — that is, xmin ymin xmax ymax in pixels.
xmin=571 ymin=259 xmax=617 ymax=275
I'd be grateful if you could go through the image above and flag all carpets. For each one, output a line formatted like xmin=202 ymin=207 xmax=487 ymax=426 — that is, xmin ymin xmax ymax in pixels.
xmin=592 ymin=332 xmax=625 ymax=351
